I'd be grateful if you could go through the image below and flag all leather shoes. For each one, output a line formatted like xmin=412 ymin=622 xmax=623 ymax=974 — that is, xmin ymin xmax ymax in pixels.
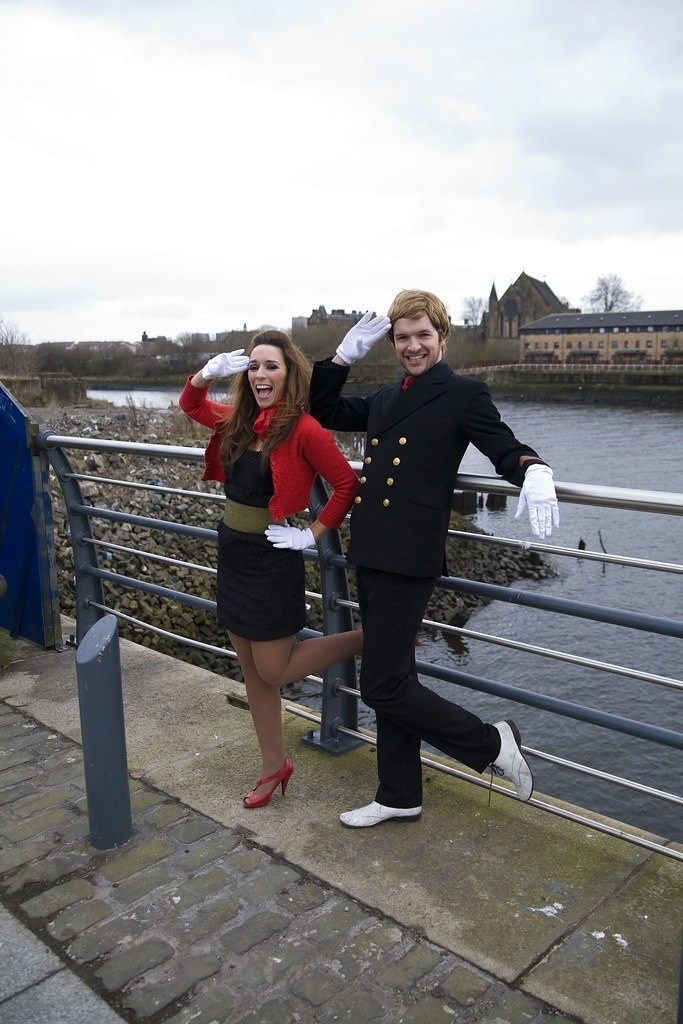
xmin=339 ymin=801 xmax=422 ymax=829
xmin=488 ymin=719 xmax=534 ymax=807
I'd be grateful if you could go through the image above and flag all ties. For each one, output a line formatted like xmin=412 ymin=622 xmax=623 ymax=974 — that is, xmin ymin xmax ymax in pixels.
xmin=402 ymin=375 xmax=415 ymax=392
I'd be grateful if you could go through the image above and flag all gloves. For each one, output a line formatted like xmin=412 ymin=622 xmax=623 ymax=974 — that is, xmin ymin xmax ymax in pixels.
xmin=515 ymin=464 xmax=559 ymax=540
xmin=335 ymin=311 xmax=392 ymax=365
xmin=201 ymin=349 xmax=250 ymax=380
xmin=264 ymin=519 xmax=316 ymax=551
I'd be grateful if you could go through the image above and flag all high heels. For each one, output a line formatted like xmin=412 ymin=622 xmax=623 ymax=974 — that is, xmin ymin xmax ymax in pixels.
xmin=242 ymin=756 xmax=293 ymax=808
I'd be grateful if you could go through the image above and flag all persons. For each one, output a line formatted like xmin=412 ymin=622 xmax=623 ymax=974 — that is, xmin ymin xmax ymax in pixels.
xmin=307 ymin=290 xmax=560 ymax=827
xmin=179 ymin=330 xmax=420 ymax=809
